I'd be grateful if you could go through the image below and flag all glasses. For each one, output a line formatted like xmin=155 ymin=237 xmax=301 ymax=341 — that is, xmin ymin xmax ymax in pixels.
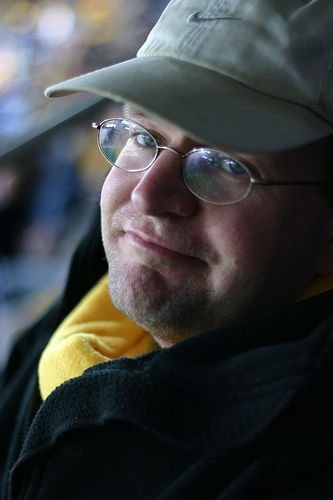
xmin=91 ymin=118 xmax=333 ymax=206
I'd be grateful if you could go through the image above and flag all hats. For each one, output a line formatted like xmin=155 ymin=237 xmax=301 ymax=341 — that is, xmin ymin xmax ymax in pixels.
xmin=45 ymin=0 xmax=333 ymax=154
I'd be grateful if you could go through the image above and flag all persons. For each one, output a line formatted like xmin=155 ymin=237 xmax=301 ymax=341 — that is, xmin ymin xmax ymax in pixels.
xmin=0 ymin=0 xmax=333 ymax=500
